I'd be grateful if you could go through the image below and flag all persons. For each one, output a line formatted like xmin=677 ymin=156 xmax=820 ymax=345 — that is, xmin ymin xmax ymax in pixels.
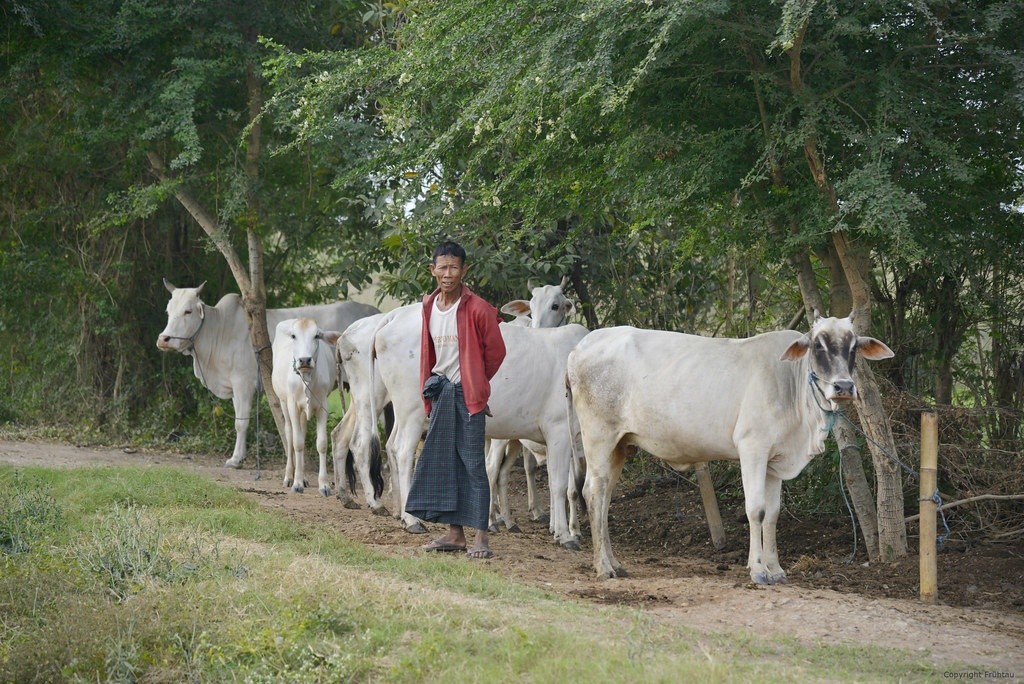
xmin=403 ymin=242 xmax=506 ymax=559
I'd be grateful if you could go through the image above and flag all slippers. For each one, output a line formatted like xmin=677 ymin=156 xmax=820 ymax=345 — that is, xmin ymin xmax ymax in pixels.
xmin=421 ymin=538 xmax=467 ymax=553
xmin=466 ymin=547 xmax=495 ymax=560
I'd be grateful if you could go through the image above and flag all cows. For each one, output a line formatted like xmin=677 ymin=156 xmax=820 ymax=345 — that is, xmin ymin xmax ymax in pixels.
xmin=154 ymin=274 xmax=384 ymax=493
xmin=564 ymin=309 xmax=895 ymax=587
xmin=331 ymin=272 xmax=588 ymax=551
